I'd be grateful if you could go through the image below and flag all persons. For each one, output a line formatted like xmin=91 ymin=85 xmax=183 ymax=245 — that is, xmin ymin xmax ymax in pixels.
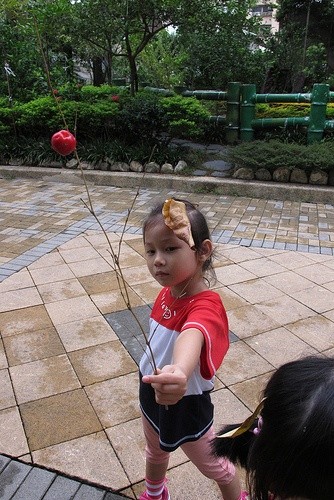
xmin=137 ymin=196 xmax=249 ymax=500
xmin=210 ymin=357 xmax=334 ymax=500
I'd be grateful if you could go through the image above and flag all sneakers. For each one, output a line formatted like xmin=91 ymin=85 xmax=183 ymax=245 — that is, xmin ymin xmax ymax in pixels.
xmin=140 ymin=486 xmax=170 ymax=500
xmin=234 ymin=490 xmax=248 ymax=499
